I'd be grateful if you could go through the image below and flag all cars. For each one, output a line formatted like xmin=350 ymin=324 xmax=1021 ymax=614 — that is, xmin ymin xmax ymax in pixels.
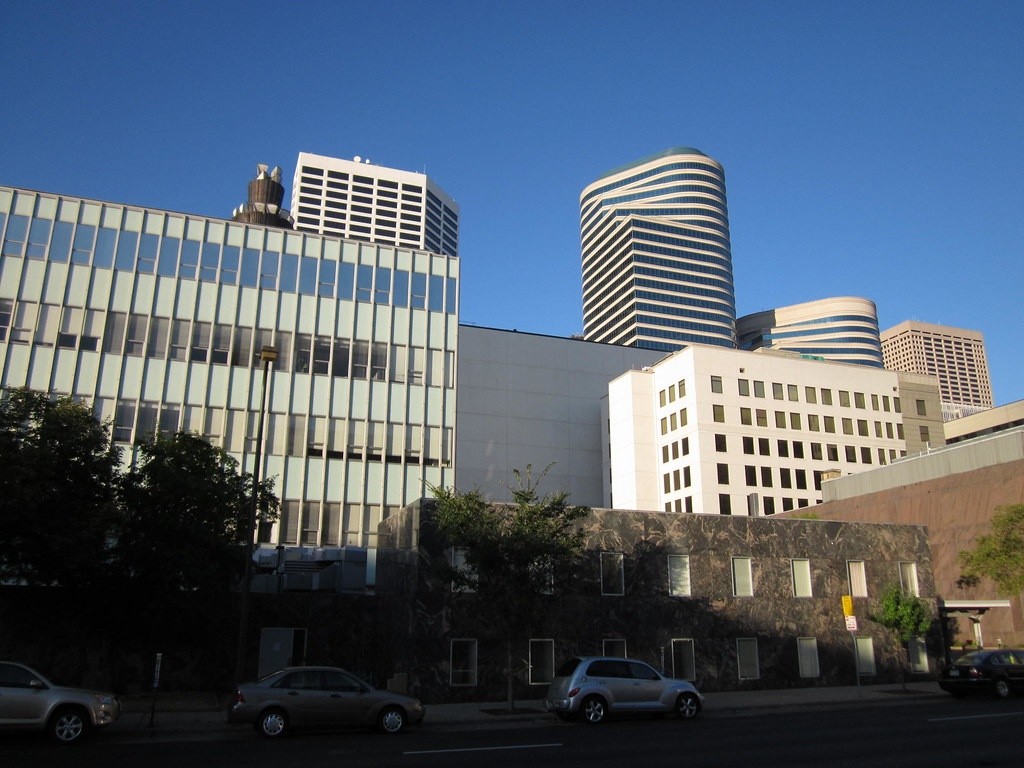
xmin=235 ymin=666 xmax=426 ymax=738
xmin=938 ymin=644 xmax=1024 ymax=697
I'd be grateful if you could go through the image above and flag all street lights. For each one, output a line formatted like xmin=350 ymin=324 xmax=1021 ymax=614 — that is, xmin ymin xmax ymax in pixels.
xmin=236 ymin=344 xmax=280 ymax=684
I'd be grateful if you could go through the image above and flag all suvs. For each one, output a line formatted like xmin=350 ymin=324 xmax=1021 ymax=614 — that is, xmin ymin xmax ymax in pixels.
xmin=0 ymin=659 xmax=122 ymax=744
xmin=544 ymin=653 xmax=706 ymax=726
